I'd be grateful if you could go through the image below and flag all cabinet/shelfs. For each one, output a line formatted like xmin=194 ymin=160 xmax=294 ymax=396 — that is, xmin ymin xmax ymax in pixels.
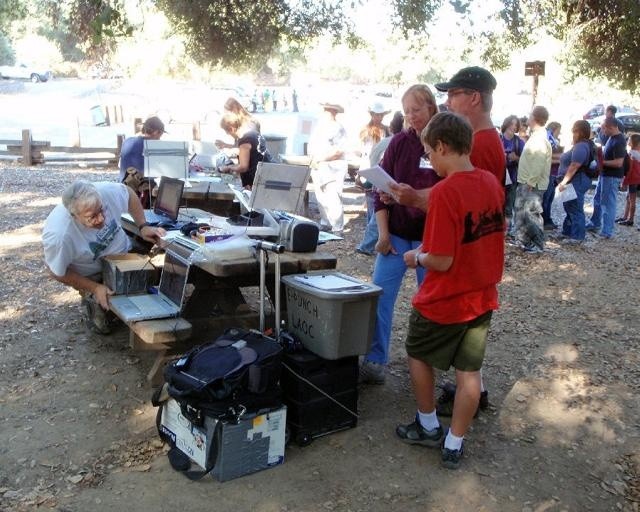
xmin=256 ymin=333 xmax=360 ymax=446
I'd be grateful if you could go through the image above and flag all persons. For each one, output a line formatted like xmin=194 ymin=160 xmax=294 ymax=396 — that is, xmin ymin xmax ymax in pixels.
xmin=375 ymin=67 xmax=507 ymax=417
xmin=118 ymin=116 xmax=166 ymax=208
xmin=217 ymin=114 xmax=282 ymax=193
xmin=308 ymin=97 xmax=404 ymax=254
xmin=394 ymin=113 xmax=506 ymax=470
xmin=355 ymin=83 xmax=445 ymax=387
xmin=215 ymin=96 xmax=262 ymax=162
xmin=41 ymin=177 xmax=167 ymax=339
xmin=498 ymin=104 xmax=640 ymax=253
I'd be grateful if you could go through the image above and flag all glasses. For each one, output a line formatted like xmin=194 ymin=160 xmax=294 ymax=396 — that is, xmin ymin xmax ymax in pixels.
xmin=83 ymin=204 xmax=103 ymax=223
xmin=446 ymin=90 xmax=467 ymax=99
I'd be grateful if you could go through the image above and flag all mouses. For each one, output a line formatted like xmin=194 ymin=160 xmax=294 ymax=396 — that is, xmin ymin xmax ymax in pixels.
xmin=157 ymin=222 xmax=175 ymax=227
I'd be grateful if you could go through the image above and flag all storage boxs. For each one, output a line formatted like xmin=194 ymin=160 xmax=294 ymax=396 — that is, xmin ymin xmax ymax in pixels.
xmin=102 ymin=252 xmax=155 ymax=297
xmin=283 ymin=270 xmax=385 ymax=362
xmin=158 ymin=388 xmax=291 ymax=484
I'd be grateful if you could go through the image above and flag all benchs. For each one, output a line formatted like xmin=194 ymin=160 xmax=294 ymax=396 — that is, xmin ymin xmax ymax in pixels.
xmin=80 ymin=276 xmax=195 ymax=347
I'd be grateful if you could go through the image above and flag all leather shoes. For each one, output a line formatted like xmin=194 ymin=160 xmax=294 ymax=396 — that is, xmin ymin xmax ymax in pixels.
xmin=615 ymin=217 xmax=625 ymax=223
xmin=618 ymin=219 xmax=634 ymax=226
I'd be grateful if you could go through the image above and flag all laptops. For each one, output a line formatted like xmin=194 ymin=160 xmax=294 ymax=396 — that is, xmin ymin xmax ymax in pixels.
xmin=122 ymin=176 xmax=184 ymax=227
xmin=107 ymin=248 xmax=192 ymax=323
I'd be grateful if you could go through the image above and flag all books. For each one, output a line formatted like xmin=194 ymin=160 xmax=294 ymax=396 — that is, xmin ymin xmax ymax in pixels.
xmin=296 ymin=274 xmax=363 ymax=291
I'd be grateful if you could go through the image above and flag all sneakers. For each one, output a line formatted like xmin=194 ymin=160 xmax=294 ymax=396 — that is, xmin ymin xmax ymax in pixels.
xmin=396 ymin=411 xmax=443 ymax=448
xmin=508 ymin=239 xmax=530 ymax=251
xmin=438 ymin=401 xmax=479 ymax=419
xmin=440 ymin=424 xmax=464 ymax=469
xmin=524 ymin=244 xmax=545 ymax=254
xmin=357 ymin=361 xmax=385 ymax=389
xmin=543 ymin=221 xmax=582 ymax=245
xmin=584 ymin=221 xmax=601 ymax=231
xmin=599 ymin=232 xmax=611 ymax=240
xmin=87 ymin=295 xmax=113 ymax=335
xmin=440 ymin=390 xmax=488 ymax=409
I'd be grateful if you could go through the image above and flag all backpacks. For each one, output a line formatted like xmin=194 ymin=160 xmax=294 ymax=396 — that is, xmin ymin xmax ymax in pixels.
xmin=579 ymin=139 xmax=603 ymax=180
xmin=167 ymin=325 xmax=284 ymax=399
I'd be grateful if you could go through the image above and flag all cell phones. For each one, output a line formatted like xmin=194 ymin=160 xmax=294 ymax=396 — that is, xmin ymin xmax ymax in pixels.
xmin=505 ymin=151 xmax=511 ymax=161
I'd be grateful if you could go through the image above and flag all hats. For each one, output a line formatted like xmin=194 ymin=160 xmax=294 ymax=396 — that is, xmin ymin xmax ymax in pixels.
xmin=369 ymin=103 xmax=391 ymax=116
xmin=434 ymin=66 xmax=496 ymax=92
xmin=140 ymin=117 xmax=169 ymax=135
xmin=319 ymin=101 xmax=345 ymax=113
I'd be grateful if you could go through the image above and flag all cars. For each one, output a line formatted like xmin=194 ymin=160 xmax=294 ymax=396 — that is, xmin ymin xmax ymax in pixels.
xmin=147 ymin=76 xmax=255 ymax=127
xmin=0 ymin=62 xmax=50 ymax=83
xmin=349 ymin=67 xmax=382 ymax=88
xmin=579 ymin=103 xmax=640 ymax=136
xmin=251 ymin=70 xmax=291 ymax=87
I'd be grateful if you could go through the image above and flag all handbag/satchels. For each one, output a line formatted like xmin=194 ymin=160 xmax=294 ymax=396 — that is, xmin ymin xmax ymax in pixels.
xmin=152 ymin=356 xmax=282 ymax=478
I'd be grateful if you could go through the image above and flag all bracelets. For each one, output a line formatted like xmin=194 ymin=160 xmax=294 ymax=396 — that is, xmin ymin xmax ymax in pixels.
xmin=138 ymin=222 xmax=148 ymax=230
xmin=414 ymin=253 xmax=422 ymax=267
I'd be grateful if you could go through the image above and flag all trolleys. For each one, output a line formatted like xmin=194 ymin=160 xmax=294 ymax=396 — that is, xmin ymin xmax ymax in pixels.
xmin=254 ymin=239 xmax=360 ymax=446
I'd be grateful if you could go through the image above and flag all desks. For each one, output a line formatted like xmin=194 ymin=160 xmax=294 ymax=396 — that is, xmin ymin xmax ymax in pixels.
xmin=178 ymin=179 xmax=241 ymax=214
xmin=279 ymin=152 xmax=368 ymax=218
xmin=119 ymin=203 xmax=338 ymax=386
